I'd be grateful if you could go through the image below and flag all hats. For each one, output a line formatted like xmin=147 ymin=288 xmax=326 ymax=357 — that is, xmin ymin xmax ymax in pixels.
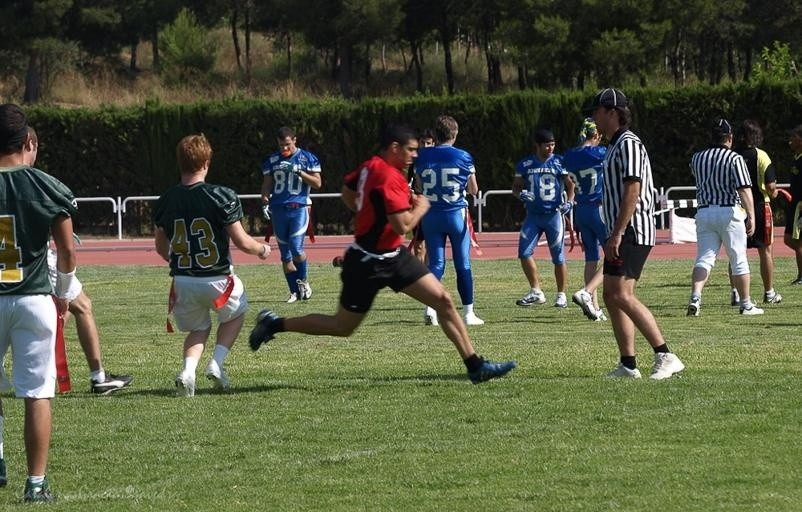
xmin=582 ymin=88 xmax=629 ymax=112
xmin=709 ymin=118 xmax=732 ymax=136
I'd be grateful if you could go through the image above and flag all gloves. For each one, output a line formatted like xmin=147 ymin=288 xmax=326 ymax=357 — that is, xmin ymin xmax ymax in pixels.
xmin=260 ymin=203 xmax=272 ymax=221
xmin=560 ymin=201 xmax=575 ymax=215
xmin=519 ymin=191 xmax=536 ymax=202
xmin=279 ymin=161 xmax=302 ymax=175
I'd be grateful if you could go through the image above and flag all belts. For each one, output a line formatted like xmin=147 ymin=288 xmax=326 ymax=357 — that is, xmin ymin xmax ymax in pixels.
xmin=697 ymin=204 xmax=736 ymax=209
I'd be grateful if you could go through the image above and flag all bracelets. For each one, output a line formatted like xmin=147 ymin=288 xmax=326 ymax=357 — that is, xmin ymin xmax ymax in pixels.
xmin=54 ymin=269 xmax=76 ymax=299
xmin=259 ymin=245 xmax=266 ymax=257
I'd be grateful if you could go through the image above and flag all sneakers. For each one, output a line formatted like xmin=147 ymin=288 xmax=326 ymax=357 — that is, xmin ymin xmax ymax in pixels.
xmin=515 ymin=291 xmax=547 ymax=306
xmin=174 ymin=370 xmax=196 ymax=397
xmin=606 ymin=362 xmax=641 ymax=380
xmin=296 ymin=278 xmax=314 ymax=300
xmin=248 ymin=308 xmax=279 ymax=353
xmin=553 ymin=294 xmax=568 ymax=308
xmin=204 ymin=358 xmax=229 ymax=390
xmin=790 ymin=277 xmax=802 ymax=285
xmin=286 ymin=292 xmax=302 ymax=304
xmin=424 ymin=307 xmax=439 ymax=327
xmin=571 ymin=288 xmax=608 ymax=323
xmin=468 ymin=359 xmax=515 ymax=386
xmin=648 ymin=351 xmax=685 ymax=380
xmin=739 ymin=305 xmax=765 ymax=316
xmin=731 ymin=289 xmax=741 ymax=306
xmin=461 ymin=311 xmax=484 ymax=326
xmin=686 ymin=295 xmax=701 ymax=317
xmin=762 ymin=291 xmax=783 ymax=304
xmin=90 ymin=370 xmax=133 ymax=396
xmin=24 ymin=476 xmax=55 ymax=503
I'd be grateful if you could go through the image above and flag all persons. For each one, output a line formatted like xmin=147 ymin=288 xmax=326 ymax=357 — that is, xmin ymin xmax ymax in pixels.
xmin=407 ymin=128 xmax=436 ymax=265
xmin=581 ymin=88 xmax=686 ymax=382
xmin=784 ymin=127 xmax=802 ymax=286
xmin=1 ymin=104 xmax=75 ymax=503
xmin=416 ymin=116 xmax=485 ymax=326
xmin=260 ymin=127 xmax=322 ymax=303
xmin=249 ymin=126 xmax=516 ymax=384
xmin=512 ymin=130 xmax=575 ymax=309
xmin=687 ymin=118 xmax=764 ymax=316
xmin=566 ymin=117 xmax=612 ymax=322
xmin=729 ymin=120 xmax=783 ymax=305
xmin=155 ymin=134 xmax=271 ymax=397
xmin=27 ymin=128 xmax=134 ymax=398
